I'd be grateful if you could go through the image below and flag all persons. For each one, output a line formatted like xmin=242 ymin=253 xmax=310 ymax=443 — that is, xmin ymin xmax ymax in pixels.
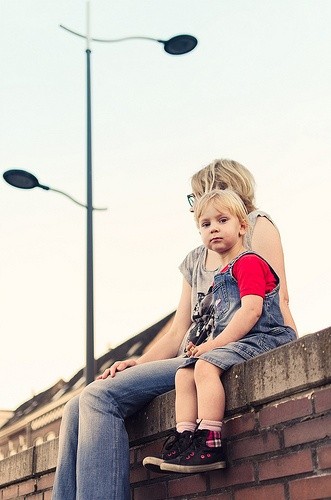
xmin=50 ymin=161 xmax=298 ymax=500
xmin=142 ymin=189 xmax=297 ymax=476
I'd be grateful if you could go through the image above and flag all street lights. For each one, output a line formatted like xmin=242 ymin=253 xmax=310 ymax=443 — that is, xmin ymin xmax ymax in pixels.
xmin=2 ymin=0 xmax=198 ymax=386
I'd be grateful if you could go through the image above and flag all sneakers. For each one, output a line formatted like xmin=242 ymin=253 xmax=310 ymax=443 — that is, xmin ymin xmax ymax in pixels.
xmin=160 ymin=420 xmax=227 ymax=473
xmin=143 ymin=426 xmax=194 ymax=473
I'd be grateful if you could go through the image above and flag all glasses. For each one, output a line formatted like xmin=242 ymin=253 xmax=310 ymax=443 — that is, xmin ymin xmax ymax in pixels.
xmin=187 ymin=193 xmax=196 ymax=207
